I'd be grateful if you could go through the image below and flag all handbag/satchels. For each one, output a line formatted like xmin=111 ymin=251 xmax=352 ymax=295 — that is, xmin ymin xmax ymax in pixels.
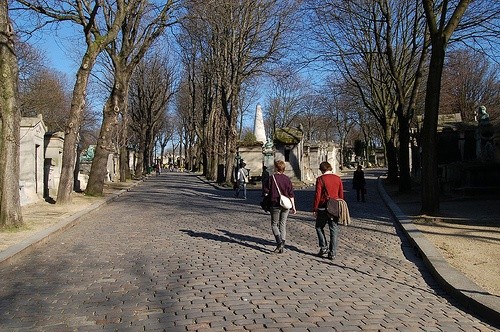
xmin=325 ymin=197 xmax=341 ymax=219
xmin=245 ymin=177 xmax=249 ymax=183
xmin=364 ymin=184 xmax=367 ymax=193
xmin=279 ymin=195 xmax=293 ymax=209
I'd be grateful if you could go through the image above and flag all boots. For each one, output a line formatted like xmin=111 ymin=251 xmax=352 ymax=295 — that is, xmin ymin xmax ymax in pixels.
xmin=274 ymin=235 xmax=286 ymax=253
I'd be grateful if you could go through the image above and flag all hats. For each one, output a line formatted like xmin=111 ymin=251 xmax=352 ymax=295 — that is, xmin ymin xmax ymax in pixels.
xmin=240 ymin=159 xmax=244 ymax=162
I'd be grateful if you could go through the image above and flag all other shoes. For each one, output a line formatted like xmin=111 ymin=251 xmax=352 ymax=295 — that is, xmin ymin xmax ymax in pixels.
xmin=319 ymin=248 xmax=327 ymax=254
xmin=328 ymin=253 xmax=336 ymax=260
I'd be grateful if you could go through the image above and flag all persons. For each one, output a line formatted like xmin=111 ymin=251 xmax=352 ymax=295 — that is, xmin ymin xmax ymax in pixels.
xmin=352 ymin=165 xmax=367 ymax=202
xmin=233 ymin=159 xmax=248 ymax=199
xmin=312 ymin=161 xmax=344 ymax=260
xmin=261 ymin=166 xmax=269 ymax=197
xmin=156 ymin=162 xmax=161 ymax=175
xmin=261 ymin=160 xmax=297 ymax=253
